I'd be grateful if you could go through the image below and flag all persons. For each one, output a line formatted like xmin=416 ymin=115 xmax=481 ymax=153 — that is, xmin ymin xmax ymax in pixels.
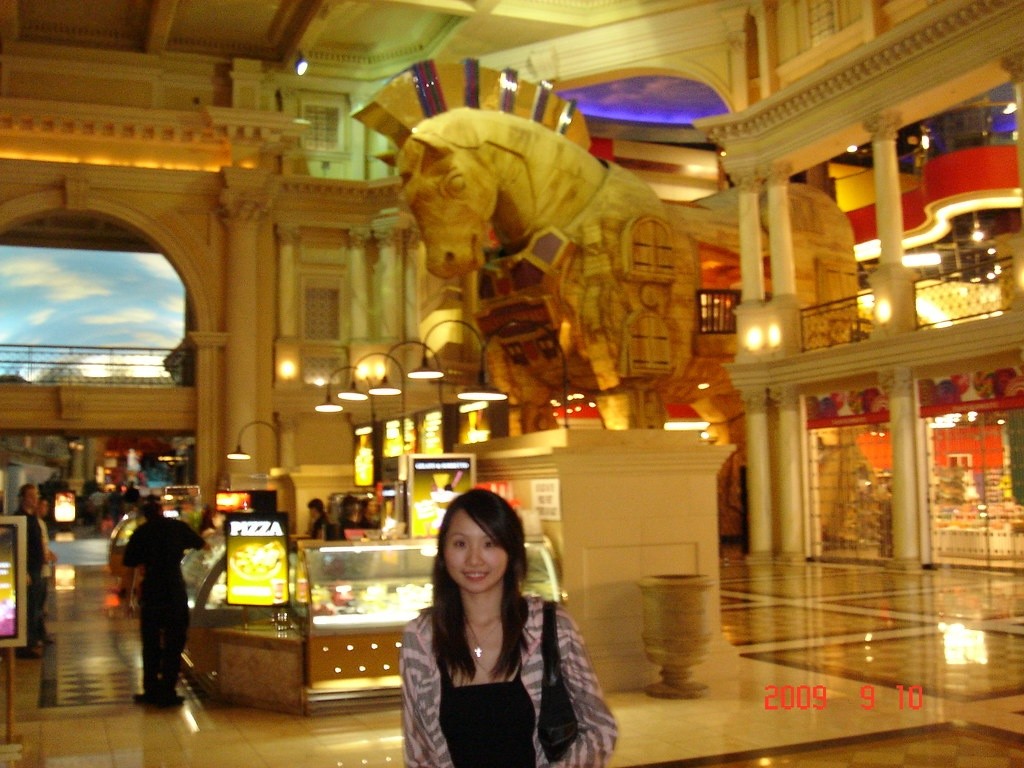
xmin=101 ymin=481 xmax=141 ymax=523
xmin=12 ymin=482 xmax=58 ymax=659
xmin=122 ymin=503 xmax=211 ymax=705
xmin=400 ymin=489 xmax=619 ymax=768
xmin=337 ymin=496 xmax=367 ymax=540
xmin=307 ymin=499 xmax=335 ymax=539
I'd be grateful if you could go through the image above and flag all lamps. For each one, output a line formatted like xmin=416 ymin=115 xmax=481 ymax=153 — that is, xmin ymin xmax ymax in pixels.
xmin=295 ymin=51 xmax=308 ymax=76
xmin=457 ymin=319 xmax=569 ymax=429
xmin=228 ymin=420 xmax=280 ymax=470
xmin=407 ymin=320 xmax=485 ymax=391
xmin=315 ymin=366 xmax=377 ymax=445
xmin=338 ymin=352 xmax=407 ymax=453
xmin=368 ymin=340 xmax=446 ymax=453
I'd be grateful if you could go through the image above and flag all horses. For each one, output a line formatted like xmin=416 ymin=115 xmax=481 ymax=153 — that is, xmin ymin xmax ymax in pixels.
xmin=350 ymin=58 xmax=746 ymax=445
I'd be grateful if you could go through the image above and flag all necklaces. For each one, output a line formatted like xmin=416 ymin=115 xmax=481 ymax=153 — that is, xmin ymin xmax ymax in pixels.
xmin=467 ymin=619 xmax=501 ymax=658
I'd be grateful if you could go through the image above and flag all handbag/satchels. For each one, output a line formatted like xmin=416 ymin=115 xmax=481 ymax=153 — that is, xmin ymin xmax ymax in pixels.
xmin=539 ymin=601 xmax=580 ymax=762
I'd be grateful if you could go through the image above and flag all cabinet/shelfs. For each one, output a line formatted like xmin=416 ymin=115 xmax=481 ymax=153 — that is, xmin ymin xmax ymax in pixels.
xmin=294 ymin=535 xmax=568 ymax=720
xmin=180 ymin=530 xmax=281 ymax=703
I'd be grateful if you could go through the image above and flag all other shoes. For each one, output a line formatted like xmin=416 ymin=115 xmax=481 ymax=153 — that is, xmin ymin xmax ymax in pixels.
xmin=165 ymin=695 xmax=186 ymax=706
xmin=133 ymin=693 xmax=152 ymax=704
